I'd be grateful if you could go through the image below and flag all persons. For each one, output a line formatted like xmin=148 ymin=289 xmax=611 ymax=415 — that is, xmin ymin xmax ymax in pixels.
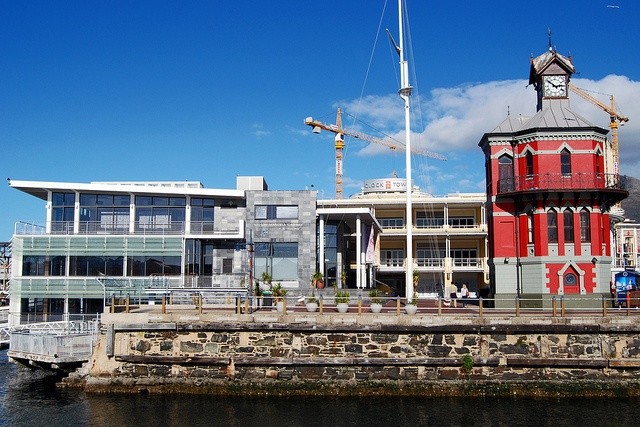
xmin=461 ymin=284 xmax=469 ymax=308
xmin=449 ymin=281 xmax=458 ymax=307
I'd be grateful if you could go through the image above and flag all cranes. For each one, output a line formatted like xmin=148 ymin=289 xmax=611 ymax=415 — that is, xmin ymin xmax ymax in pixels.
xmin=568 ymin=81 xmax=629 ymax=187
xmin=304 ymin=106 xmax=448 ymax=199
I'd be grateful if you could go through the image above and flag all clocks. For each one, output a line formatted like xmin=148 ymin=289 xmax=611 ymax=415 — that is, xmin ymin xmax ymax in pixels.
xmin=541 ymin=74 xmax=569 ymax=99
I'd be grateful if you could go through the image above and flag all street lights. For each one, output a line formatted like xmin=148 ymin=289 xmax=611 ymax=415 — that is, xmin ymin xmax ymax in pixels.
xmin=244 ymin=241 xmax=254 ymax=312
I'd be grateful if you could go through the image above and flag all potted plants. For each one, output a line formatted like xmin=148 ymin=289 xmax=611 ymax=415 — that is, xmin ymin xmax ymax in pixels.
xmin=332 ymin=281 xmax=350 ymax=313
xmin=254 ymin=271 xmax=272 ymax=296
xmin=305 ymin=295 xmax=319 ymax=312
xmin=311 ymin=272 xmax=324 ymax=289
xmin=369 ymin=288 xmax=383 ymax=313
xmin=405 ymin=292 xmax=418 ymax=314
xmin=272 ymin=283 xmax=289 ymax=312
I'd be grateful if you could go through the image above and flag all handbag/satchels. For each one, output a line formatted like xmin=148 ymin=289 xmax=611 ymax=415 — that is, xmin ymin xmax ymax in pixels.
xmin=466 ymin=289 xmax=469 ymax=297
